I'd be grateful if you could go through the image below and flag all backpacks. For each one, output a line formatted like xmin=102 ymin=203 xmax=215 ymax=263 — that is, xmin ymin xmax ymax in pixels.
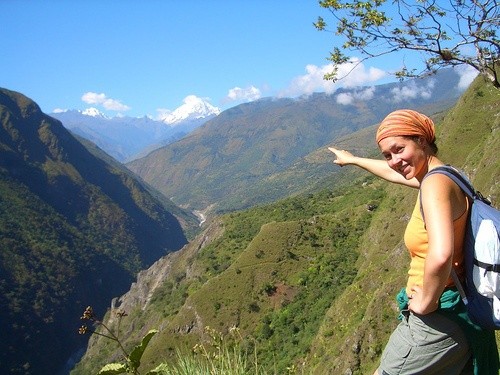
xmin=418 ymin=163 xmax=500 ymax=331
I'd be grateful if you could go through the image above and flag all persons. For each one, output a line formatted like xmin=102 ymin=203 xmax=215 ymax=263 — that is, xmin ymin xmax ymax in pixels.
xmin=327 ymin=109 xmax=500 ymax=375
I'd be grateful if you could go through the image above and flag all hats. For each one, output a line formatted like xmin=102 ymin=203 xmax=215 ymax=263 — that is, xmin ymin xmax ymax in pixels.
xmin=375 ymin=109 xmax=435 ymax=144
